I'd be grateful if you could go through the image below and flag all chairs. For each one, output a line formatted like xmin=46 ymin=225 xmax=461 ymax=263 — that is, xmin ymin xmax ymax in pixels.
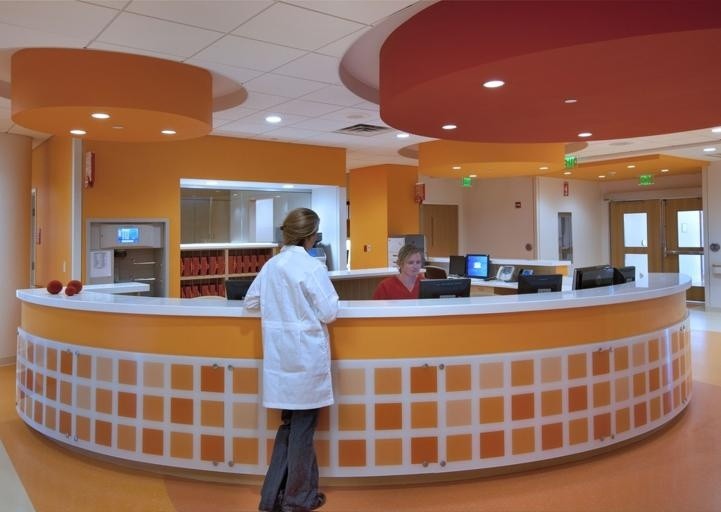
xmin=424 ymin=266 xmax=447 ymax=279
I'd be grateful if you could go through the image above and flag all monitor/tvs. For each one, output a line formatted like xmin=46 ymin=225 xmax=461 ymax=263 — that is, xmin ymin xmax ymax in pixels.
xmin=466 ymin=254 xmax=489 ymax=278
xmin=518 ymin=275 xmax=562 ymax=295
xmin=225 ymin=280 xmax=254 ymax=300
xmin=449 ymin=256 xmax=466 ymax=276
xmin=419 ymin=279 xmax=471 ymax=299
xmin=572 ymin=265 xmax=614 ymax=290
xmin=613 ymin=266 xmax=635 ymax=285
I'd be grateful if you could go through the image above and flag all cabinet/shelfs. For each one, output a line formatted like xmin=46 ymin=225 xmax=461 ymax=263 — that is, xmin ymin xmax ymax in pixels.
xmin=179 ymin=242 xmax=279 ymax=300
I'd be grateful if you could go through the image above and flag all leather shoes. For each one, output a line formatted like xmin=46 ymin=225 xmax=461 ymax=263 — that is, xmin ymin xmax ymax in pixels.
xmin=312 ymin=492 xmax=326 ymax=510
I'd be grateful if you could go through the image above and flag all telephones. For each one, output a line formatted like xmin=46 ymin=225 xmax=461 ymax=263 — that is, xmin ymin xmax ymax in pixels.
xmin=496 ymin=265 xmax=516 ymax=281
xmin=519 ymin=269 xmax=534 ymax=275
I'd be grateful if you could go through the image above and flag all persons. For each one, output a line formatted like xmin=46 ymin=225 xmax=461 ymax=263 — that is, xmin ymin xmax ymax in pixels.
xmin=373 ymin=245 xmax=427 ymax=299
xmin=243 ymin=208 xmax=339 ymax=512
